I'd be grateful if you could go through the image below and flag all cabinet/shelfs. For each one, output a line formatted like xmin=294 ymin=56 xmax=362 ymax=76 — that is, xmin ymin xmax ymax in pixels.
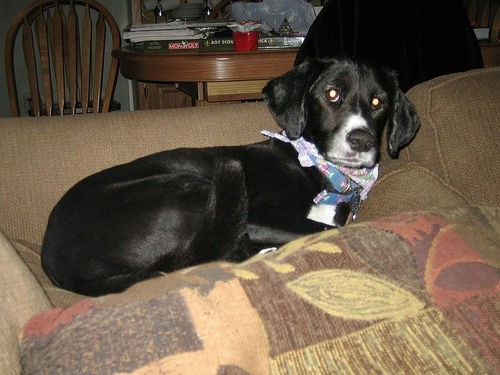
xmin=111 ymin=46 xmax=299 ymax=110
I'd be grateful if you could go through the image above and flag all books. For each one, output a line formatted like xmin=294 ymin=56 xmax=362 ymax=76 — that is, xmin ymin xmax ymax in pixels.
xmin=122 ymin=20 xmax=220 ymax=42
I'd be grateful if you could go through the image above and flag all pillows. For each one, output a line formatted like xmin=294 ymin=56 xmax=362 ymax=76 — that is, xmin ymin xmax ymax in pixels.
xmin=18 ymin=205 xmax=500 ymax=374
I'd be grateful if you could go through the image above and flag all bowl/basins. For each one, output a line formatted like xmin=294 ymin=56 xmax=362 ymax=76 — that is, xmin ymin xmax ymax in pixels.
xmin=231 ymin=31 xmax=260 ymax=50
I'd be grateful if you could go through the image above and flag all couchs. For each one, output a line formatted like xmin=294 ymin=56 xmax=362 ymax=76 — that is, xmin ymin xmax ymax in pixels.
xmin=0 ymin=67 xmax=500 ymax=373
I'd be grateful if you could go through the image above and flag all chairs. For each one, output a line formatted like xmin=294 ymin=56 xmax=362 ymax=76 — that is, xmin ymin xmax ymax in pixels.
xmin=0 ymin=0 xmax=121 ymax=118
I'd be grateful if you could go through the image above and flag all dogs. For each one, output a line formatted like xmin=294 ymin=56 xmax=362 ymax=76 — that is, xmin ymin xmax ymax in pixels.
xmin=39 ymin=53 xmax=422 ymax=297
